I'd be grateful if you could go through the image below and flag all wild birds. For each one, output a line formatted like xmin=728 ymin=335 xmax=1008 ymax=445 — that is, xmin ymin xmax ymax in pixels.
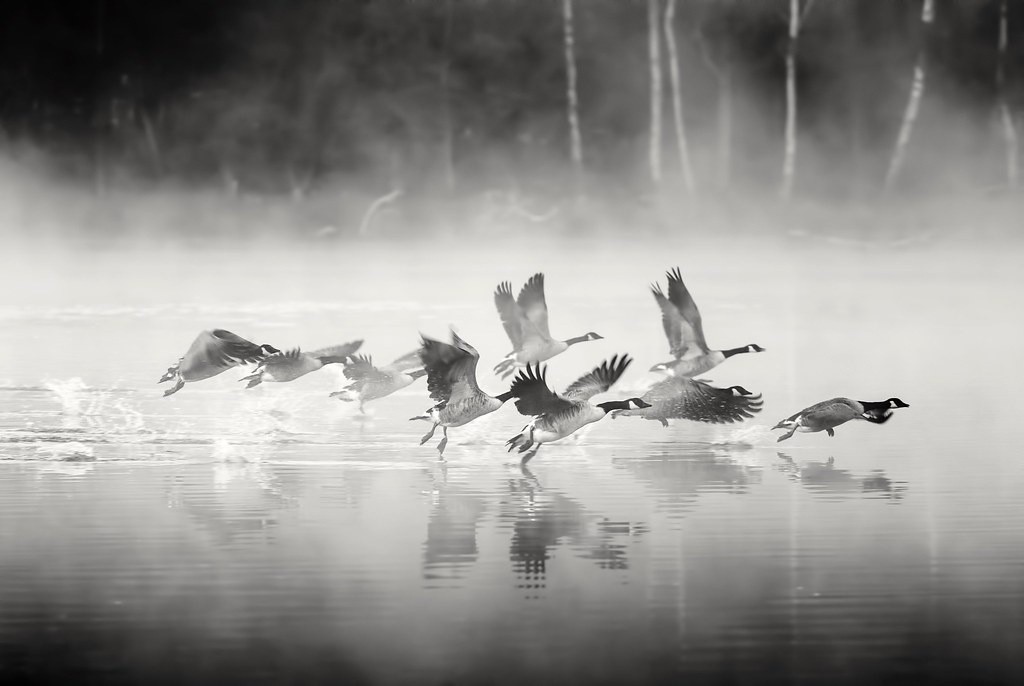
xmin=493 ymin=273 xmax=606 ymax=381
xmin=646 ymin=265 xmax=768 ymax=379
xmin=772 ymin=397 xmax=910 ymax=442
xmin=159 ymin=327 xmax=766 ymax=464
